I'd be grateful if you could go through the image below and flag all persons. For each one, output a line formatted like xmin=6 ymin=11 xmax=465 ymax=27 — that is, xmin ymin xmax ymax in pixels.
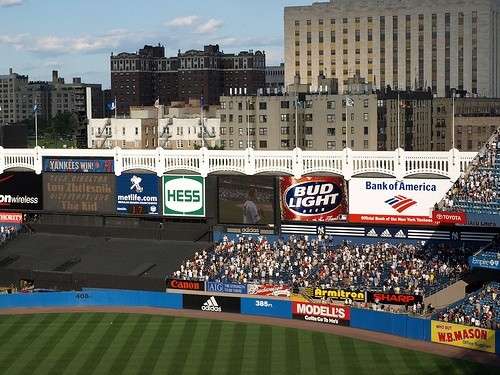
xmin=1 ymin=211 xmax=40 ymax=245
xmin=25 ymin=281 xmax=35 ymax=293
xmin=171 ymin=128 xmax=499 ymax=330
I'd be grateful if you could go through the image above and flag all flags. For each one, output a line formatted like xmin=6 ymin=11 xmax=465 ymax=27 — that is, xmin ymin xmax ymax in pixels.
xmin=247 ymin=96 xmax=253 ymax=105
xmin=32 ymin=104 xmax=37 ymax=113
xmin=154 ymin=99 xmax=159 ymax=110
xmin=345 ymin=96 xmax=355 ymax=106
xmin=398 ymin=96 xmax=407 ymax=110
xmin=106 ymin=102 xmax=116 ymax=110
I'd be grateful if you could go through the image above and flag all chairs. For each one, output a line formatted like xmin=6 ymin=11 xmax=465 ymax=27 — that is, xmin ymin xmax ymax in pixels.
xmin=442 ymin=144 xmax=500 ymax=215
xmin=443 ymin=282 xmax=500 ymax=323
xmin=474 ymin=249 xmax=500 ymax=268
